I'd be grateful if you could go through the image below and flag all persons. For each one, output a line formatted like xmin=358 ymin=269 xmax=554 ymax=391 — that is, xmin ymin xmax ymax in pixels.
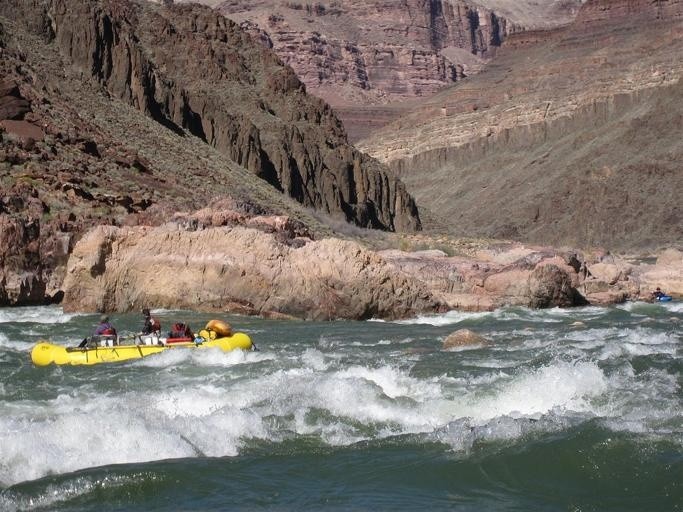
xmin=652 ymin=287 xmax=664 ymax=297
xmin=96 ymin=314 xmax=117 ymax=337
xmin=140 ymin=309 xmax=155 ymax=335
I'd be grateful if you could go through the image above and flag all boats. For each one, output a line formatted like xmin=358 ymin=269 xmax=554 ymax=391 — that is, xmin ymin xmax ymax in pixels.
xmin=28 ymin=332 xmax=253 ymax=367
